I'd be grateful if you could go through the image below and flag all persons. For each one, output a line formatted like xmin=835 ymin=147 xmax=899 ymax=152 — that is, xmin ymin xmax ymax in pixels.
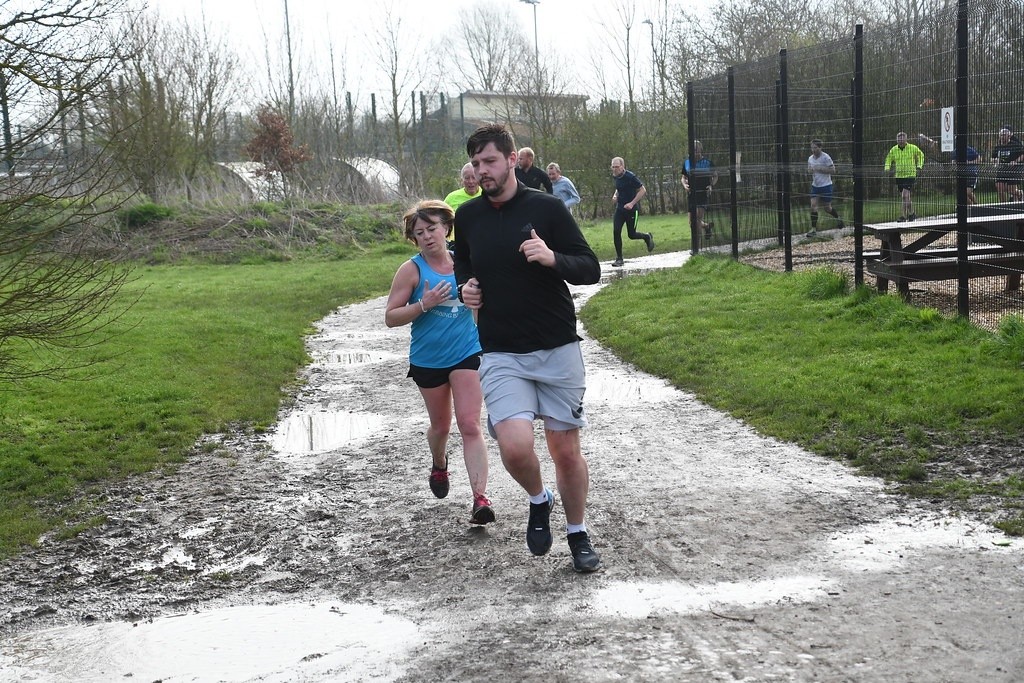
xmin=385 ymin=200 xmax=495 ymax=526
xmin=454 ymin=125 xmax=601 ymax=573
xmin=884 ymin=132 xmax=924 ymax=222
xmin=991 ymin=125 xmax=1024 ymax=203
xmin=681 ymin=140 xmax=718 ymax=255
xmin=806 ymin=139 xmax=843 ymax=236
xmin=514 ymin=147 xmax=553 ymax=195
xmin=610 ymin=157 xmax=655 ymax=267
xmin=444 ymin=163 xmax=482 ymax=252
xmin=951 ymin=146 xmax=980 ymax=204
xmin=547 ymin=162 xmax=580 ymax=212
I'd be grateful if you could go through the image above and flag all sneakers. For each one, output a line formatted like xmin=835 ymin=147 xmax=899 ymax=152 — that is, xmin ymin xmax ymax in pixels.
xmin=525 ymin=487 xmax=555 ymax=557
xmin=429 ymin=456 xmax=450 ymax=499
xmin=470 ymin=492 xmax=495 ymax=525
xmin=566 ymin=531 xmax=599 ymax=572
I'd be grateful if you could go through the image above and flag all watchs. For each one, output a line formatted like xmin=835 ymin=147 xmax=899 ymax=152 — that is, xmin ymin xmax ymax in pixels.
xmin=458 ymin=284 xmax=464 ymax=303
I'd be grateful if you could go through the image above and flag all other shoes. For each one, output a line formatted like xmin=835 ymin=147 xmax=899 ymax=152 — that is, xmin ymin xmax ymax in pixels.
xmin=1018 ymin=190 xmax=1023 ymax=201
xmin=908 ymin=213 xmax=915 ymax=222
xmin=897 ymin=216 xmax=906 ymax=222
xmin=612 ymin=258 xmax=624 ymax=266
xmin=704 ymin=222 xmax=714 ymax=240
xmin=645 ymin=232 xmax=654 ymax=252
xmin=806 ymin=230 xmax=817 ymax=237
xmin=689 ymin=251 xmax=693 ymax=255
xmin=837 ymin=215 xmax=843 ymax=229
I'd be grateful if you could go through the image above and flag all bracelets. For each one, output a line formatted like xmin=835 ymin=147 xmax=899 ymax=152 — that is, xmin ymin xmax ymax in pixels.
xmin=418 ymin=299 xmax=427 ymax=312
xmin=711 ymin=184 xmax=713 ymax=187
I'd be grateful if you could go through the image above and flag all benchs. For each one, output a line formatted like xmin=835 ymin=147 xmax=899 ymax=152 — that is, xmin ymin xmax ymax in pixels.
xmin=862 ymin=245 xmax=1024 ymax=282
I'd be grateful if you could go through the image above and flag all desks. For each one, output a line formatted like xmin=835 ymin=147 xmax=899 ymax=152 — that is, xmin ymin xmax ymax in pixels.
xmin=862 ymin=213 xmax=1024 ymax=300
